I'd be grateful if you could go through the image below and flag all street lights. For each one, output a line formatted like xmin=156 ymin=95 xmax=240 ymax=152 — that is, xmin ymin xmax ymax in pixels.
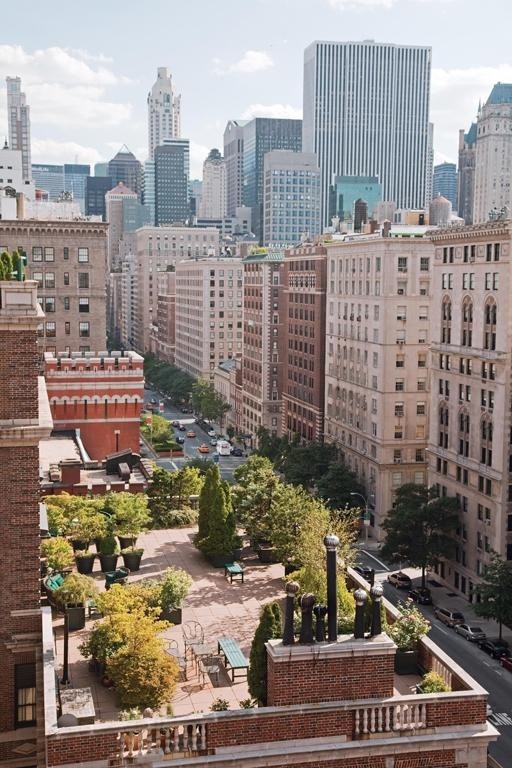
xmin=486 ymin=596 xmax=502 ymax=639
xmin=349 ymin=491 xmax=375 ymax=543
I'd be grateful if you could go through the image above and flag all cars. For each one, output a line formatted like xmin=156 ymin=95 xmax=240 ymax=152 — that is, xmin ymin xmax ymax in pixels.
xmin=139 ymin=382 xmax=253 ymax=463
xmin=486 ymin=702 xmax=493 ymax=717
xmin=499 ymin=647 xmax=512 ymax=671
xmin=453 ymin=622 xmax=486 ymax=642
xmin=477 ymin=637 xmax=509 ymax=659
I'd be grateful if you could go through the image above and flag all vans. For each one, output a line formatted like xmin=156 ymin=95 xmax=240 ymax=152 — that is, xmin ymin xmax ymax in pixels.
xmin=354 ymin=565 xmax=375 ymax=587
xmin=434 ymin=604 xmax=464 ymax=628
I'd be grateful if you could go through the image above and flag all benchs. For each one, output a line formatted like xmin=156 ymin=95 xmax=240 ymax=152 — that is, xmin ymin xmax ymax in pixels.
xmin=217 ymin=636 xmax=251 ymax=683
xmin=224 ymin=560 xmax=244 ymax=584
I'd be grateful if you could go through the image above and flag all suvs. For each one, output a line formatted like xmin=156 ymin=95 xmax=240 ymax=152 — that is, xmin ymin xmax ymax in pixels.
xmin=407 ymin=586 xmax=432 ymax=605
xmin=387 ymin=572 xmax=411 ymax=589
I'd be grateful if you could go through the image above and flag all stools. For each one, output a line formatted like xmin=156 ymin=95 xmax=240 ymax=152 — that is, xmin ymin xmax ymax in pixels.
xmin=88 ymin=601 xmax=104 ymax=619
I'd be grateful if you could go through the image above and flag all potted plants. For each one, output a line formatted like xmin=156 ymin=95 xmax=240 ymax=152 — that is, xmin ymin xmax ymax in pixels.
xmin=155 ymin=568 xmax=192 ymax=625
xmin=56 ymin=573 xmax=94 ymax=630
xmin=117 ymin=705 xmax=145 ymax=749
xmin=416 ymin=670 xmax=452 ymax=711
xmin=194 ymin=462 xmax=285 ymax=567
xmin=46 ymin=489 xmax=144 ymax=573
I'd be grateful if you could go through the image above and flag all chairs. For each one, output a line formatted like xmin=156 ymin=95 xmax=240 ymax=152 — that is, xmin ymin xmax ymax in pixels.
xmin=157 ymin=618 xmax=221 ymax=688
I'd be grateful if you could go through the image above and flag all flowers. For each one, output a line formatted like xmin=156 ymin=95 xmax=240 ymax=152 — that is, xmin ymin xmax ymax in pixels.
xmin=387 ymin=597 xmax=432 ymax=651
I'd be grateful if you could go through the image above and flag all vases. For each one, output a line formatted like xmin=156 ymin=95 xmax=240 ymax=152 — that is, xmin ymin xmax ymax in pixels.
xmin=394 ymin=649 xmax=421 ymax=676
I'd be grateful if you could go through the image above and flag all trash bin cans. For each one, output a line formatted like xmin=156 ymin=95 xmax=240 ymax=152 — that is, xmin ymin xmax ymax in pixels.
xmin=395 ymin=647 xmax=419 ymax=675
